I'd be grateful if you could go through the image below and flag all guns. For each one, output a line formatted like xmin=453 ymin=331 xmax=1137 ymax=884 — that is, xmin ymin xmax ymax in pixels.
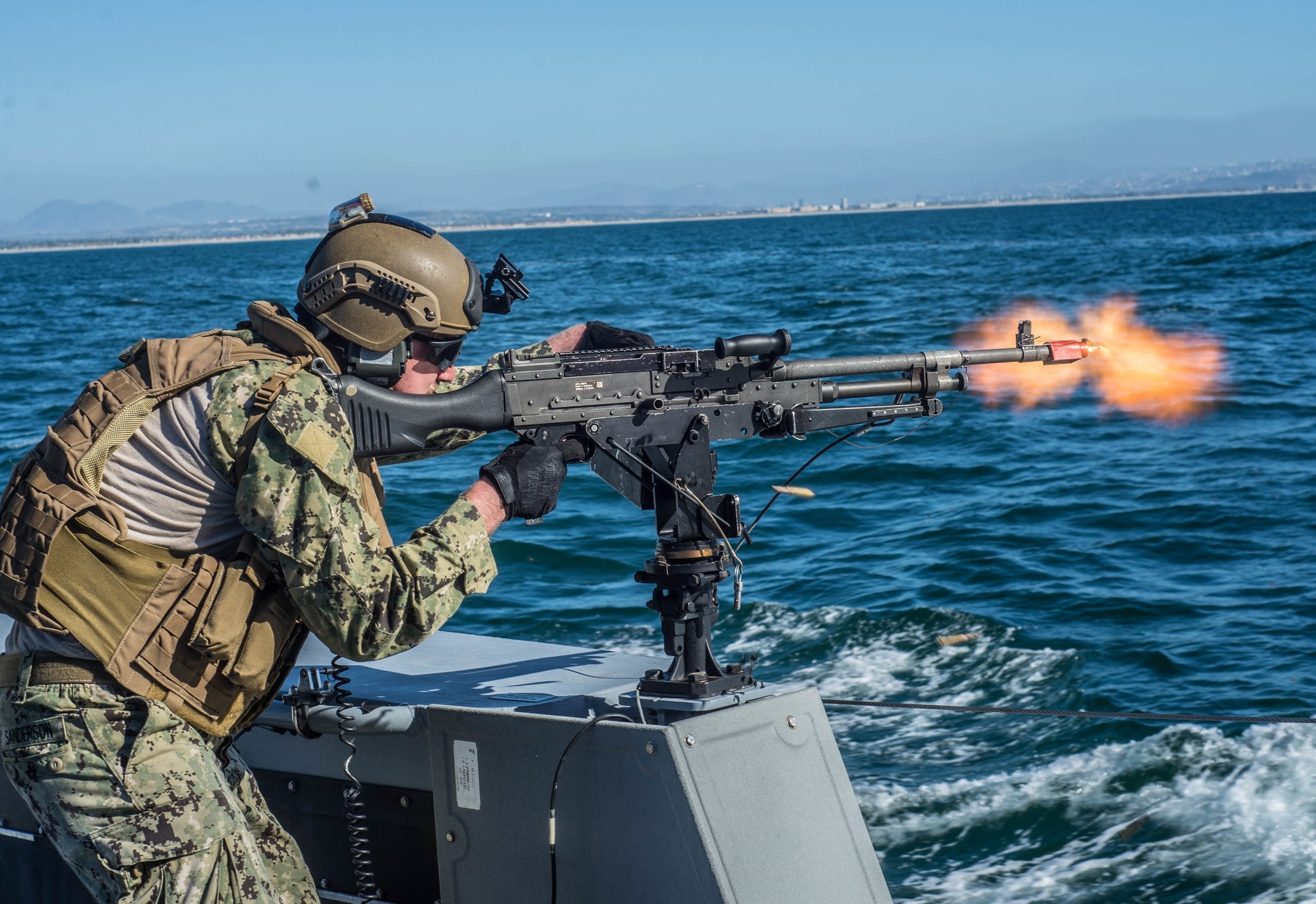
xmin=310 ymin=319 xmax=1089 ymax=465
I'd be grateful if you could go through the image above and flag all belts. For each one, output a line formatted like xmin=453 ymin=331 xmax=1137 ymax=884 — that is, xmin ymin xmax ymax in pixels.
xmin=0 ymin=653 xmax=119 ymax=685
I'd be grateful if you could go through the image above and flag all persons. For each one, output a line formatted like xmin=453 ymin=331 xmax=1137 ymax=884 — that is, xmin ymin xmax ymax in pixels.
xmin=0 ymin=212 xmax=658 ymax=904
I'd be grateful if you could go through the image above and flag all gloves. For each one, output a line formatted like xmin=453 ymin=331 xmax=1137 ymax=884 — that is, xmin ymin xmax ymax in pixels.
xmin=579 ymin=320 xmax=655 ymax=349
xmin=479 ymin=438 xmax=585 ymax=520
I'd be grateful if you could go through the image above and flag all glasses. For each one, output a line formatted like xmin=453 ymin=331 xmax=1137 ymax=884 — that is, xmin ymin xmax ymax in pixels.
xmin=411 ymin=332 xmax=466 ymax=371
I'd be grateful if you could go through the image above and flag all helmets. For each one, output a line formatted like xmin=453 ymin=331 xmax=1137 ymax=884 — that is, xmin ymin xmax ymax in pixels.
xmin=296 ymin=214 xmax=484 ymax=352
